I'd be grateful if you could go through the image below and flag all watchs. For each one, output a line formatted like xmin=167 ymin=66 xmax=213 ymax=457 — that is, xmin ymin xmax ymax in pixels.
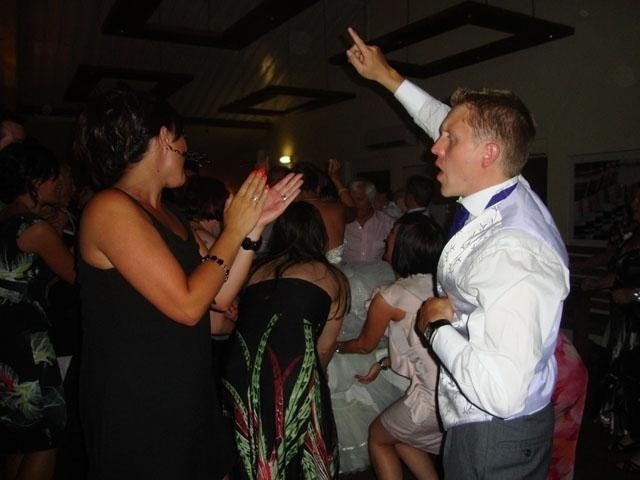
xmin=422 ymin=318 xmax=452 ymax=347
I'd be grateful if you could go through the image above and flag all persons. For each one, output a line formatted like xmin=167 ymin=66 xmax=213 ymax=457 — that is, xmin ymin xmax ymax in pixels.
xmin=70 ymin=86 xmax=308 ymax=479
xmin=570 ymin=177 xmax=640 ymax=480
xmin=545 ymin=330 xmax=589 ymax=480
xmin=334 ymin=211 xmax=445 ymax=479
xmin=0 ymin=134 xmax=78 ymax=479
xmin=344 ymin=27 xmax=571 ymax=480
xmin=1 ymin=119 xmax=433 ymax=378
xmin=214 ymin=199 xmax=351 ymax=480
